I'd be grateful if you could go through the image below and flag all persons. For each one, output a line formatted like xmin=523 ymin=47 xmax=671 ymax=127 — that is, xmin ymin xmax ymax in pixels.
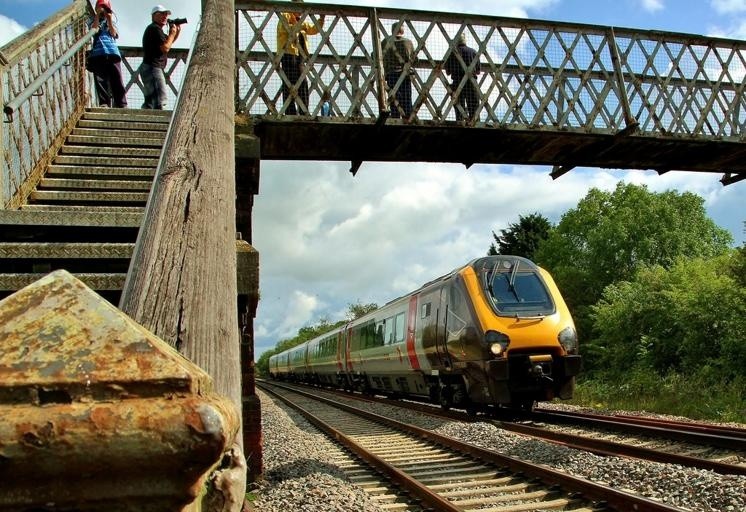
xmin=87 ymin=0 xmax=128 ymax=108
xmin=139 ymin=4 xmax=181 ymax=109
xmin=444 ymin=33 xmax=481 ymax=122
xmin=276 ymin=0 xmax=326 ymax=116
xmin=370 ymin=21 xmax=419 ymax=119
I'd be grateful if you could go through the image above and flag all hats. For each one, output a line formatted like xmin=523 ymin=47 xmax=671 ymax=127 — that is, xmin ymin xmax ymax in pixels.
xmin=95 ymin=0 xmax=112 ymax=10
xmin=151 ymin=4 xmax=171 ymax=16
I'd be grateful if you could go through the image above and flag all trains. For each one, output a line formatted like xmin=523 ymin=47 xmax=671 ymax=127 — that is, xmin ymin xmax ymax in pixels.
xmin=268 ymin=254 xmax=580 ymax=416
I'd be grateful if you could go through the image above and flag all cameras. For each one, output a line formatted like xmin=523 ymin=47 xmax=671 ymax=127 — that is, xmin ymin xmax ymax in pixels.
xmin=166 ymin=18 xmax=187 ymax=26
xmin=102 ymin=5 xmax=112 ymax=13
